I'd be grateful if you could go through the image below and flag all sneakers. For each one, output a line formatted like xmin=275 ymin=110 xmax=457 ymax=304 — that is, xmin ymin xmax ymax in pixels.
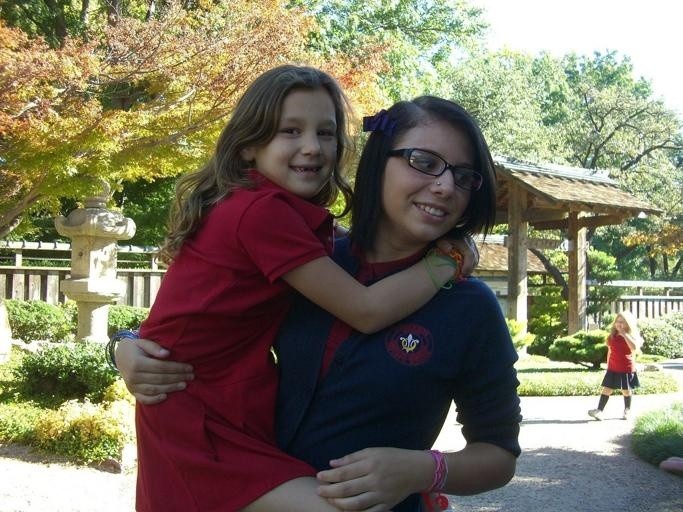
xmin=588 ymin=409 xmax=604 ymax=421
xmin=623 ymin=410 xmax=631 ymax=420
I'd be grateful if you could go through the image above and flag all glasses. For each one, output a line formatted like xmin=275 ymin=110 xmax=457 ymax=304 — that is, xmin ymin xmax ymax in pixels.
xmin=388 ymin=148 xmax=483 ymax=192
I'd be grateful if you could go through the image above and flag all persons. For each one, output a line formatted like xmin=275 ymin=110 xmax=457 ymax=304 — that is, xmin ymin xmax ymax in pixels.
xmin=588 ymin=311 xmax=641 ymax=420
